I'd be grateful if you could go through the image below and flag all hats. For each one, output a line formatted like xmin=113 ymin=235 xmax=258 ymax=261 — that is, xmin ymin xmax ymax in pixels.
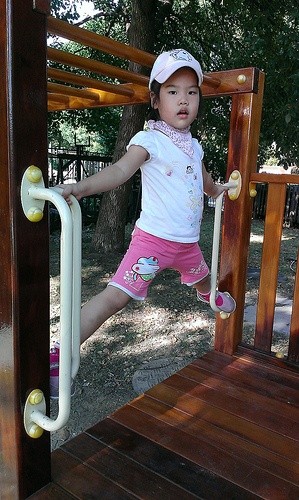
xmin=148 ymin=48 xmax=203 ymax=93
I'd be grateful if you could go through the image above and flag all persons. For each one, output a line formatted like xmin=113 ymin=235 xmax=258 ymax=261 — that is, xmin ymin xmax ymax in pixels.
xmin=49 ymin=48 xmax=236 ymax=400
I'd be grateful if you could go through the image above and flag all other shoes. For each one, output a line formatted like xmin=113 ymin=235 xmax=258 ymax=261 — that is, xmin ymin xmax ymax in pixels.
xmin=197 ymin=288 xmax=236 ymax=313
xmin=46 ymin=344 xmax=75 ymax=400
